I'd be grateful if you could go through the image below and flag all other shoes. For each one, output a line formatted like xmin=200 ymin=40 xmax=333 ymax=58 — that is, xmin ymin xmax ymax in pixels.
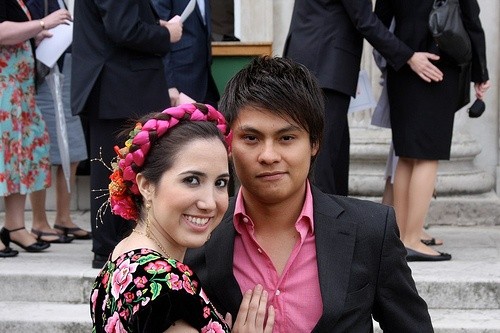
xmin=91 ymin=250 xmax=111 ymax=270
xmin=53 ymin=222 xmax=93 ymax=240
xmin=403 ymin=247 xmax=452 ymax=262
xmin=29 ymin=228 xmax=77 ymax=245
xmin=420 ymin=237 xmax=444 ymax=246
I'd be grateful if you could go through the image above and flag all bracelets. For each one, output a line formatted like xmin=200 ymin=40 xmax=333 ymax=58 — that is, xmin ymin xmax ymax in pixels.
xmin=40 ymin=19 xmax=46 ymax=30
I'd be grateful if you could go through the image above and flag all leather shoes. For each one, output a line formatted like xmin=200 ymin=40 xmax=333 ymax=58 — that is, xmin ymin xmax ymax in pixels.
xmin=0 ymin=248 xmax=18 ymax=257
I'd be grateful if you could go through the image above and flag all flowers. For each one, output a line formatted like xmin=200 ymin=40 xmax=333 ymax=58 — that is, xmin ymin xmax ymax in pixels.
xmin=89 ymin=147 xmax=139 ymax=229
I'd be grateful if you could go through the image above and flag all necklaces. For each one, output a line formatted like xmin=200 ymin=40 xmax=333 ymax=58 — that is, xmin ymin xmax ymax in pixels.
xmin=133 ymin=229 xmax=171 ymax=259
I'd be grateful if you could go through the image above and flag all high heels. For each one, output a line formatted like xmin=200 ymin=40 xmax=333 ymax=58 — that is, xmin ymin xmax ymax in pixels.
xmin=0 ymin=226 xmax=51 ymax=257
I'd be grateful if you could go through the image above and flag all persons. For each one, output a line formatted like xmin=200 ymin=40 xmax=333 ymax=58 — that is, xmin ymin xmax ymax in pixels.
xmin=0 ymin=0 xmax=491 ymax=262
xmin=89 ymin=103 xmax=275 ymax=333
xmin=182 ymin=55 xmax=435 ymax=333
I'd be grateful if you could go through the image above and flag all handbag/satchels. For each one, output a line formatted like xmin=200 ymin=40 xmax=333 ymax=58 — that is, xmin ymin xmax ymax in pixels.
xmin=428 ymin=0 xmax=473 ymax=64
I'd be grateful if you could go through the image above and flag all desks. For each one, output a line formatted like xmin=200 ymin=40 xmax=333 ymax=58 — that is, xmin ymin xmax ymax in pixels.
xmin=210 ymin=41 xmax=272 ymax=59
